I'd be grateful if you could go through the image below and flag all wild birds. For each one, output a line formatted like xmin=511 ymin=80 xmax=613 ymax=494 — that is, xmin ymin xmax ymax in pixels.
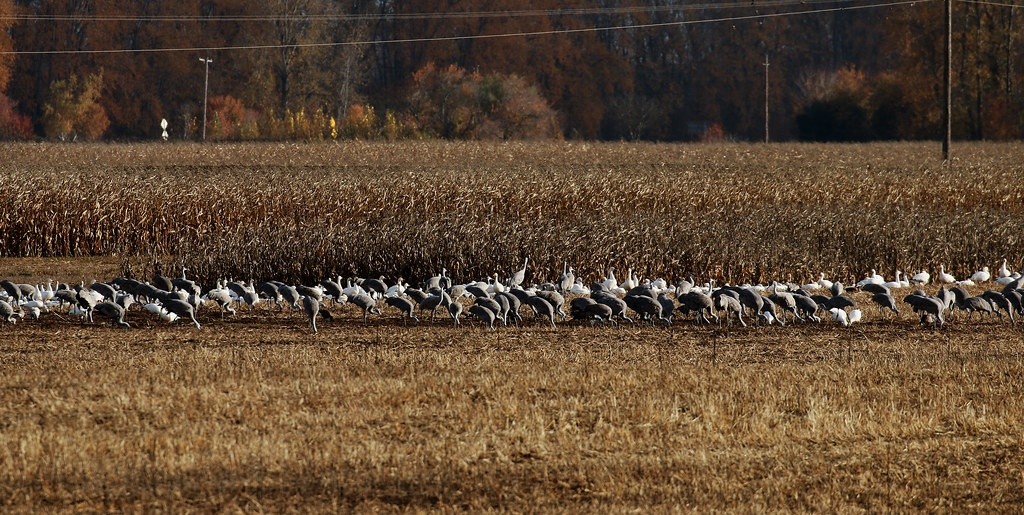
xmin=1 ymin=257 xmax=1024 ymax=333
